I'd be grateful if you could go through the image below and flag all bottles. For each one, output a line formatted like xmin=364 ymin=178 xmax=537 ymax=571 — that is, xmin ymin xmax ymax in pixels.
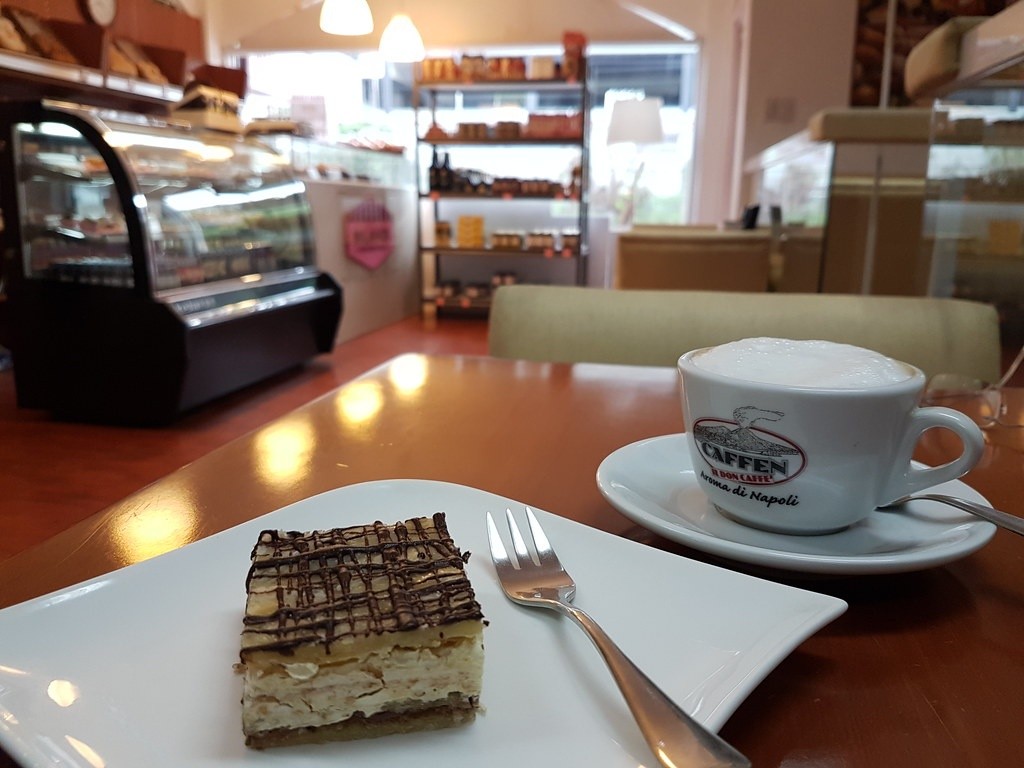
xmin=422 ymin=55 xmax=582 ymax=308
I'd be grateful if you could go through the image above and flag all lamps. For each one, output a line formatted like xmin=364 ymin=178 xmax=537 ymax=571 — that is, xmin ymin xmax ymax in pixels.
xmin=378 ymin=15 xmax=425 ymax=62
xmin=318 ymin=0 xmax=374 ymax=36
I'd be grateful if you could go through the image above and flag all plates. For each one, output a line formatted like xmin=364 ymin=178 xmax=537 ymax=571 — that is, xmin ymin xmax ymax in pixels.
xmin=596 ymin=432 xmax=997 ymax=574
xmin=0 ymin=477 xmax=854 ymax=767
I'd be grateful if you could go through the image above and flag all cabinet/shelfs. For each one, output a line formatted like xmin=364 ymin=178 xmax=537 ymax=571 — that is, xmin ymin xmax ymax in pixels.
xmin=0 ymin=0 xmax=1024 ymax=403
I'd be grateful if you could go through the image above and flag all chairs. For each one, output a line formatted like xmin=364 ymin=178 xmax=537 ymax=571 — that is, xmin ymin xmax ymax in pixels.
xmin=484 ymin=282 xmax=1001 ymax=402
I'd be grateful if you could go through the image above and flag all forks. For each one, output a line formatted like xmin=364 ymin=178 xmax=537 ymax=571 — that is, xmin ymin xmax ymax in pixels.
xmin=483 ymin=505 xmax=754 ymax=767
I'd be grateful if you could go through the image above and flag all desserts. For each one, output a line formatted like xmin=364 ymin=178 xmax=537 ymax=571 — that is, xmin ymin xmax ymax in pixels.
xmin=234 ymin=512 xmax=491 ymax=749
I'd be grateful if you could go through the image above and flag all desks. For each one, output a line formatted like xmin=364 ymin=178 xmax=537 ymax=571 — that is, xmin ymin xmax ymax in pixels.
xmin=0 ymin=346 xmax=1024 ymax=768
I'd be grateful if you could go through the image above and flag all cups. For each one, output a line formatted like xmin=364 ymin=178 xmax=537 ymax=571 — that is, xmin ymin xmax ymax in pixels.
xmin=677 ymin=347 xmax=986 ymax=538
